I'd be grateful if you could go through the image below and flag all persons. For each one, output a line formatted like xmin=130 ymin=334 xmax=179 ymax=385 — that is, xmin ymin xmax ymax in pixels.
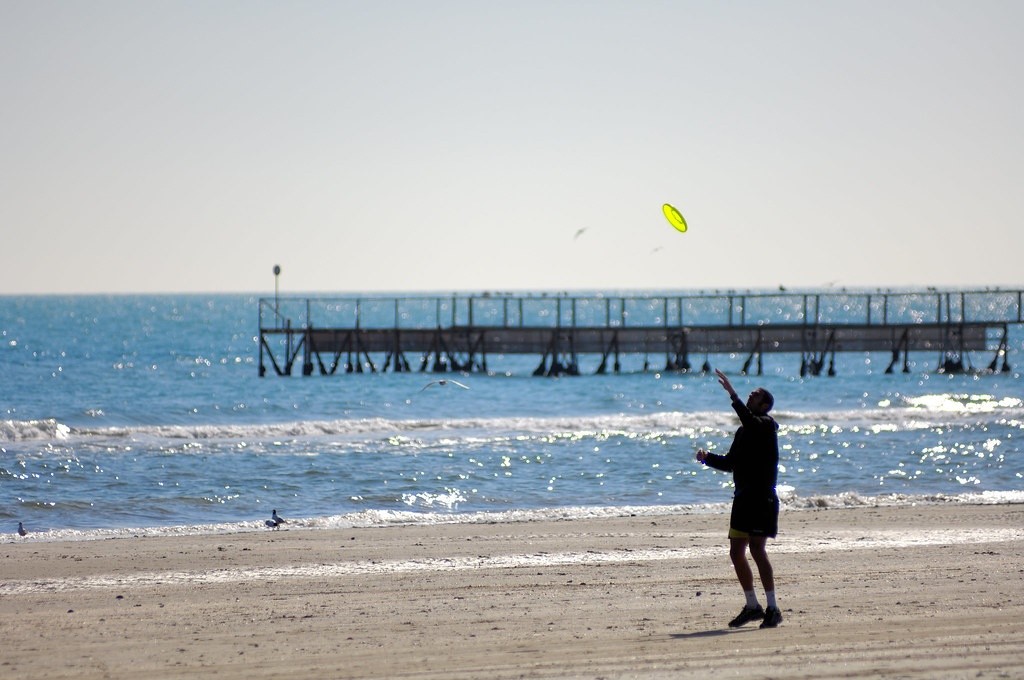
xmin=696 ymin=368 xmax=783 ymax=629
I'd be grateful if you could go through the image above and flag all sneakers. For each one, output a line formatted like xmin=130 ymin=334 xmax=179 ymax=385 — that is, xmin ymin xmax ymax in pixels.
xmin=760 ymin=606 xmax=783 ymax=629
xmin=728 ymin=602 xmax=763 ymax=628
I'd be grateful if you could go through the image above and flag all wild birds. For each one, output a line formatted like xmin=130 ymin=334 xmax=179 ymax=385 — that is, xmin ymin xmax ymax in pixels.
xmin=271 ymin=509 xmax=285 ymax=531
xmin=265 ymin=519 xmax=278 ymax=531
xmin=17 ymin=522 xmax=26 ymax=538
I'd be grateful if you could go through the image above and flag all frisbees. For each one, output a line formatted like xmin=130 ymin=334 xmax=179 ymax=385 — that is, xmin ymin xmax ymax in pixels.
xmin=662 ymin=203 xmax=688 ymax=233
